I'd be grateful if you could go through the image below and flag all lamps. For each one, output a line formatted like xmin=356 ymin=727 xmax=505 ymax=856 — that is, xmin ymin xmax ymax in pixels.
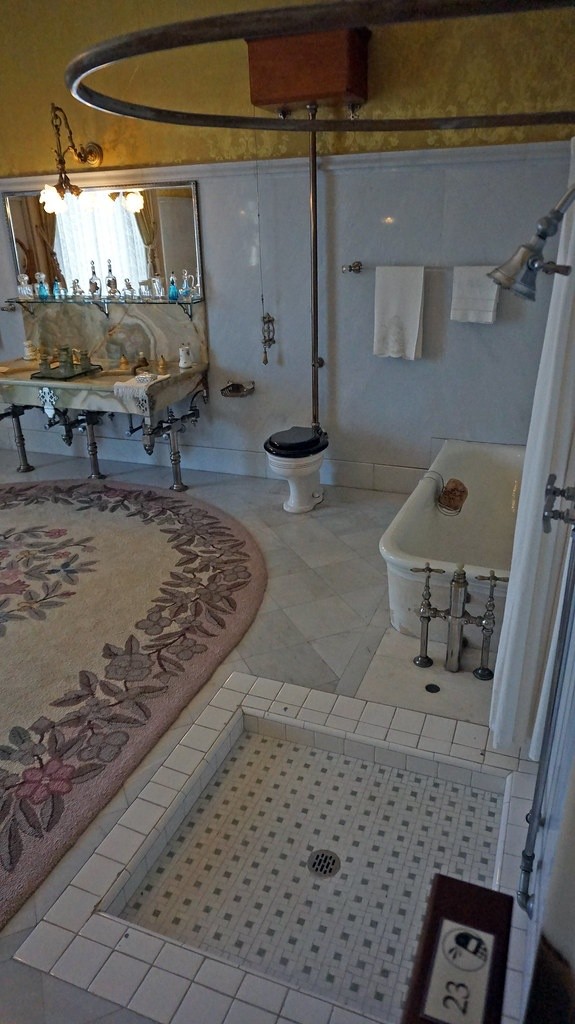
xmin=39 ymin=102 xmax=103 ymax=216
xmin=108 ymin=188 xmax=143 ymax=211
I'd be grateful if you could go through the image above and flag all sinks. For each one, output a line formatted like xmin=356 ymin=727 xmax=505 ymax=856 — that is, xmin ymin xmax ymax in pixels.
xmin=90 ymin=370 xmax=146 ymax=383
xmin=4 ymin=367 xmax=52 ymax=379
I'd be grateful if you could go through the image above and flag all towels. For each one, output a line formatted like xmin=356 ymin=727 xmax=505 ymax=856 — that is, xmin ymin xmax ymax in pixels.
xmin=448 ymin=265 xmax=504 ymax=325
xmin=113 ymin=372 xmax=172 ymax=399
xmin=372 ymin=265 xmax=427 ymax=360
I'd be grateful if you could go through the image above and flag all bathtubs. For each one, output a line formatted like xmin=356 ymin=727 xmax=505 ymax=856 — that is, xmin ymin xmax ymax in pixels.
xmin=377 ymin=438 xmax=527 ymax=653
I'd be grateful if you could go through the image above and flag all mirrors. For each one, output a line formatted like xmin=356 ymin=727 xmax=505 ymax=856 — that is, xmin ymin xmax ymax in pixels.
xmin=1 ymin=179 xmax=206 ymax=296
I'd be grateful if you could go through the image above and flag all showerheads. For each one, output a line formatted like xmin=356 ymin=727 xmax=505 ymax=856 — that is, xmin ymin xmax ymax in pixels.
xmin=485 ymin=244 xmax=545 ymax=301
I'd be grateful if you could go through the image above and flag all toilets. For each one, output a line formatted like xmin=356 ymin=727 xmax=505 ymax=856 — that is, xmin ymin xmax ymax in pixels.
xmin=263 ymin=424 xmax=329 ymax=514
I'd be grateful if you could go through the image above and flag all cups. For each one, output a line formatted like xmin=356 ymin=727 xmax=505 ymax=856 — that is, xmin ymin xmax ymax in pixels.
xmin=179 ymin=347 xmax=192 ymax=368
xmin=139 ymin=281 xmax=153 ymax=300
xmin=151 ymin=277 xmax=165 ymax=296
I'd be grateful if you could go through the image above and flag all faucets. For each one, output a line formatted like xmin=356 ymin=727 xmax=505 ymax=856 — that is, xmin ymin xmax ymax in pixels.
xmin=131 ymin=351 xmax=148 ymax=376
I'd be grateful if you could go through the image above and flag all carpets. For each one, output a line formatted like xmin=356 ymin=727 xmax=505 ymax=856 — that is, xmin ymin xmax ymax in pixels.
xmin=0 ymin=478 xmax=268 ymax=934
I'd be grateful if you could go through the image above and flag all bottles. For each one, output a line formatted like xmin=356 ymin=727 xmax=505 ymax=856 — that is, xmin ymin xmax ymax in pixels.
xmin=108 ymin=280 xmax=121 ymax=300
xmin=105 ymin=259 xmax=117 ymax=297
xmin=168 ymin=280 xmax=178 ymax=299
xmin=169 ymin=271 xmax=177 ymax=285
xmin=121 ymin=278 xmax=136 ymax=300
xmin=34 ymin=272 xmax=49 ymax=295
xmin=179 ymin=269 xmax=196 ymax=300
xmin=17 ymin=274 xmax=34 ymax=299
xmin=53 ymin=277 xmax=62 ymax=295
xmin=89 ymin=260 xmax=101 ymax=299
xmin=38 ymin=281 xmax=48 ymax=299
